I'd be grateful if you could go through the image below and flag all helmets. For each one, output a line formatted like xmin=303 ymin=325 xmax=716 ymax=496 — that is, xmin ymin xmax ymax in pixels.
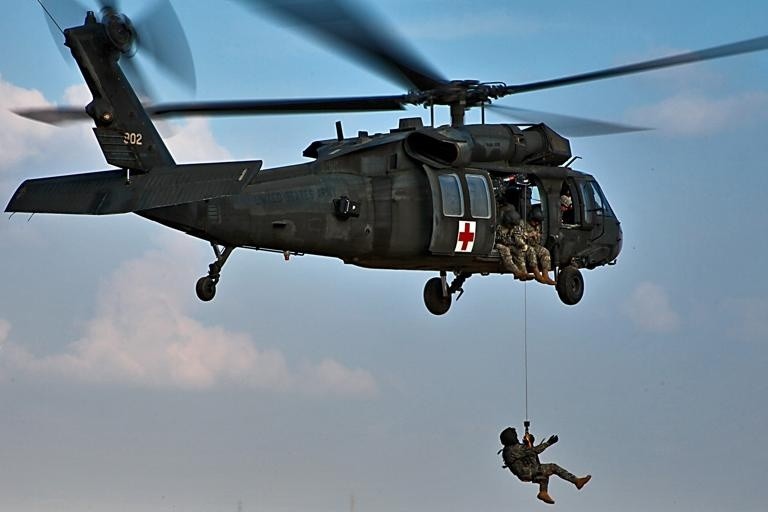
xmin=501 ymin=211 xmax=520 ymax=229
xmin=499 ymin=426 xmax=519 ymax=446
xmin=559 ymin=195 xmax=573 ymax=208
xmin=526 ymin=207 xmax=544 ymax=222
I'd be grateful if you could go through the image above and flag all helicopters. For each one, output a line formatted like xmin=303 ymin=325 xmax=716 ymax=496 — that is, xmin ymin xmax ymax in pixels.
xmin=3 ymin=0 xmax=768 ymax=316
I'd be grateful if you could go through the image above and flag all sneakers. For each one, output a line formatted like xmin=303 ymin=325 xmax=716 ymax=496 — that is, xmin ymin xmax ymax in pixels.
xmin=574 ymin=474 xmax=592 ymax=490
xmin=537 ymin=490 xmax=555 ymax=504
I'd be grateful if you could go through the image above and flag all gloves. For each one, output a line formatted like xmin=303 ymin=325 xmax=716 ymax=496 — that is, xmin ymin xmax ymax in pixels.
xmin=524 ymin=434 xmax=535 ymax=444
xmin=548 ymin=434 xmax=560 ymax=446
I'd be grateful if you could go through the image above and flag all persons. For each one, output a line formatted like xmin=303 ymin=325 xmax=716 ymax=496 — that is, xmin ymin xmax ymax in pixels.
xmin=499 ymin=426 xmax=592 ymax=506
xmin=495 ymin=210 xmax=536 ymax=282
xmin=558 ymin=193 xmax=580 ymax=230
xmin=518 ymin=210 xmax=558 ymax=287
xmin=495 ymin=180 xmax=542 ymax=233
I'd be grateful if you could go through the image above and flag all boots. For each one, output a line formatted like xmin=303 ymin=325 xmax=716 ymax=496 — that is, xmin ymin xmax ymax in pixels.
xmin=513 ymin=269 xmax=557 ymax=285
